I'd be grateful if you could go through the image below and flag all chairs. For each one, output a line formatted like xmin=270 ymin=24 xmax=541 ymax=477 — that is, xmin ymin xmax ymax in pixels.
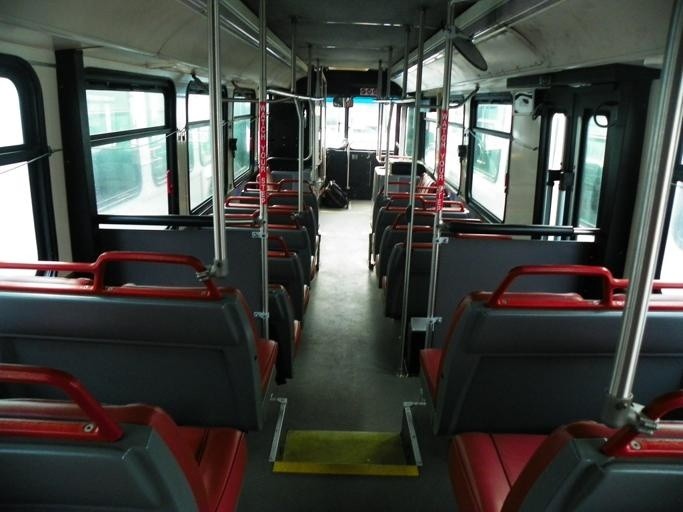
xmin=267 ymin=236 xmax=310 ymax=318
xmin=371 ymin=182 xmax=448 ymax=233
xmin=270 ymin=284 xmax=300 ymax=373
xmin=240 ymin=178 xmax=318 ymax=234
xmin=446 ymin=387 xmax=682 ymax=511
xmin=419 ymin=264 xmax=683 ymax=435
xmin=223 ymin=193 xmax=319 ymax=255
xmin=0 ymin=250 xmax=279 ymax=432
xmin=225 ymin=208 xmax=315 ymax=284
xmin=0 ymin=362 xmax=246 ymax=512
xmin=372 ymin=195 xmax=470 ymax=255
xmin=375 ymin=211 xmax=484 ymax=287
xmin=381 ymin=233 xmax=515 ymax=321
